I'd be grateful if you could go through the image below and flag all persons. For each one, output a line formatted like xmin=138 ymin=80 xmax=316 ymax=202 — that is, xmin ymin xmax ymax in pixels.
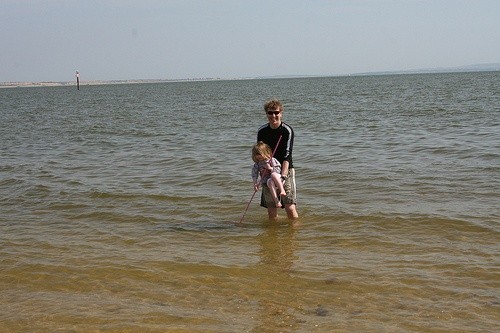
xmin=251 ymin=99 xmax=299 ymax=220
xmin=252 ymin=141 xmax=286 ymax=208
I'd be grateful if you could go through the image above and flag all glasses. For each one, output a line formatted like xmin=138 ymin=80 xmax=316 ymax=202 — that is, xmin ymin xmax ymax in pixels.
xmin=266 ymin=111 xmax=281 ymax=115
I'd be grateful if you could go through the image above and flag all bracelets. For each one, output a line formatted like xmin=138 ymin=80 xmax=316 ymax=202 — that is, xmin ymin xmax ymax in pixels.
xmin=280 ymin=176 xmax=286 ymax=184
